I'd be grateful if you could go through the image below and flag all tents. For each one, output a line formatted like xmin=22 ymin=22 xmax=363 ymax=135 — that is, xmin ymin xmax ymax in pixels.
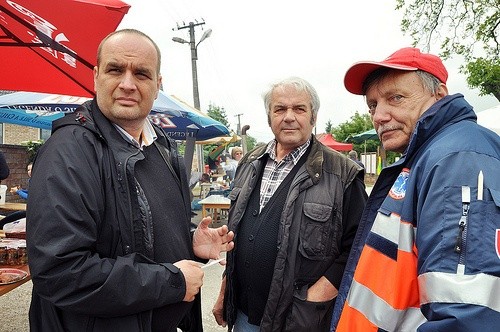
xmin=314 ymin=129 xmax=379 ymax=151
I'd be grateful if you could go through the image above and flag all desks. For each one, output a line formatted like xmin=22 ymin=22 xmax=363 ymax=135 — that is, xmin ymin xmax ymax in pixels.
xmin=0 ymin=202 xmax=26 ymax=212
xmin=198 ymin=195 xmax=231 ymax=227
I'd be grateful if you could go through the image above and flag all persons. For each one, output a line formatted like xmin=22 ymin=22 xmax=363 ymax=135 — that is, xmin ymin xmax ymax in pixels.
xmin=348 ymin=150 xmax=364 ymax=168
xmin=331 ymin=47 xmax=500 ymax=332
xmin=213 ymin=77 xmax=368 ymax=332
xmin=0 ymin=29 xmax=243 ymax=332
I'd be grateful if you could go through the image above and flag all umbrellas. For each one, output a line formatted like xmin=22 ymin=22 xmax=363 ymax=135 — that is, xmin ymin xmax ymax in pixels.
xmin=0 ymin=0 xmax=241 ymax=172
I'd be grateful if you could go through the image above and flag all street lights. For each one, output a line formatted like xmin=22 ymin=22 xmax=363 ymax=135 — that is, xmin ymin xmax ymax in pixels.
xmin=171 ymin=17 xmax=213 ymax=185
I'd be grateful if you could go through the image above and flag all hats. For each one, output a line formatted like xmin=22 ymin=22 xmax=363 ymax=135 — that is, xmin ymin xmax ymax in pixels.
xmin=344 ymin=47 xmax=449 ymax=94
